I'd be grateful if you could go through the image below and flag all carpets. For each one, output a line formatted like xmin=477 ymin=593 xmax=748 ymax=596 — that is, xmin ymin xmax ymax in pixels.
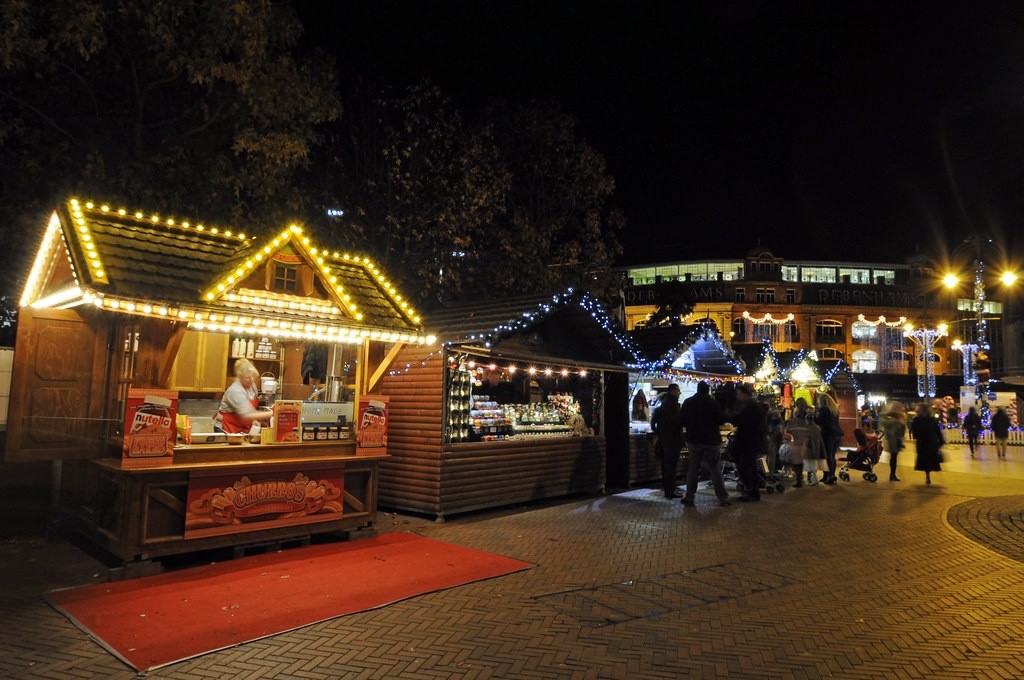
xmin=34 ymin=530 xmax=539 ymax=675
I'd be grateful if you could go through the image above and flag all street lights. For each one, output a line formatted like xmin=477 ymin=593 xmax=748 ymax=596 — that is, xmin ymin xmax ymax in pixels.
xmin=942 ymin=258 xmax=1018 ymax=427
xmin=902 ymin=321 xmax=949 ymax=416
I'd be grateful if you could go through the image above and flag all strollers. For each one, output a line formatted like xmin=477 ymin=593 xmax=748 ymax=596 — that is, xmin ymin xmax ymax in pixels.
xmin=706 ymin=431 xmax=786 ymax=495
xmin=838 ymin=427 xmax=886 ymax=482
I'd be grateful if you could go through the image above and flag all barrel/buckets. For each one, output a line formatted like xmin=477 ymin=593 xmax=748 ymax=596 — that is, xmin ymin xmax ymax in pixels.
xmin=260 ymin=372 xmax=275 ymax=392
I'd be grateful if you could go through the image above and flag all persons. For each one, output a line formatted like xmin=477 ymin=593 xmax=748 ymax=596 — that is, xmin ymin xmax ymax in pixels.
xmin=911 ymin=404 xmax=946 ymax=485
xmin=680 ymin=381 xmax=730 ymax=505
xmin=908 ymin=414 xmax=915 ymax=438
xmin=861 ymin=401 xmax=870 ymax=431
xmin=769 ymin=393 xmax=843 ymax=488
xmin=881 ymin=402 xmax=907 ymax=481
xmin=725 ymin=387 xmax=767 ymax=502
xmin=632 ymin=389 xmax=649 ymax=422
xmin=647 ymin=389 xmax=667 ymax=405
xmin=212 ymin=357 xmax=275 ymax=434
xmin=961 ymin=406 xmax=983 ymax=458
xmin=990 ymin=407 xmax=1011 ymax=458
xmin=651 ymin=383 xmax=683 ymax=498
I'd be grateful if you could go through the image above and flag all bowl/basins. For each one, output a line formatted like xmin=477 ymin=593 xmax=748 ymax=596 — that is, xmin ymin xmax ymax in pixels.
xmin=226 ymin=434 xmax=244 ymax=445
xmin=247 ymin=434 xmax=261 ymax=443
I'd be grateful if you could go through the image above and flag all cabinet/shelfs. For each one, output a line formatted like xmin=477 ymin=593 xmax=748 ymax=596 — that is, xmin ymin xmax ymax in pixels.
xmin=170 ymin=329 xmax=229 ymax=393
xmin=229 ymin=337 xmax=281 ymax=402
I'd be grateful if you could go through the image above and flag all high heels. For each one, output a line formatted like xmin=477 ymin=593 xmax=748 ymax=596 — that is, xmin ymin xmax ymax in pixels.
xmin=826 ymin=477 xmax=837 ymax=484
xmin=819 ymin=476 xmax=827 ymax=481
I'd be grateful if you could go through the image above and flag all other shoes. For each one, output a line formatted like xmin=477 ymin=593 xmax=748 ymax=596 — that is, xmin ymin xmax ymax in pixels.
xmin=721 ymin=500 xmax=731 ymax=506
xmin=890 ymin=474 xmax=900 ymax=481
xmin=681 ymin=497 xmax=693 ymax=504
xmin=792 ymin=481 xmax=802 ymax=487
xmin=665 ymin=490 xmax=683 ymax=498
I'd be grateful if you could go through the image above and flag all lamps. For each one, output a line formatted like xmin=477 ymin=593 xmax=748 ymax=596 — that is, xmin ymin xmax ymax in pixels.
xmin=465 ymin=359 xmax=589 ymax=377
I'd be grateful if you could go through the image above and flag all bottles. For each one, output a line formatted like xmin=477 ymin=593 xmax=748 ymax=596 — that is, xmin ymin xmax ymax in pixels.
xmin=247 ymin=340 xmax=254 ymax=358
xmin=239 ymin=339 xmax=246 ymax=357
xmin=232 ymin=338 xmax=240 ymax=357
